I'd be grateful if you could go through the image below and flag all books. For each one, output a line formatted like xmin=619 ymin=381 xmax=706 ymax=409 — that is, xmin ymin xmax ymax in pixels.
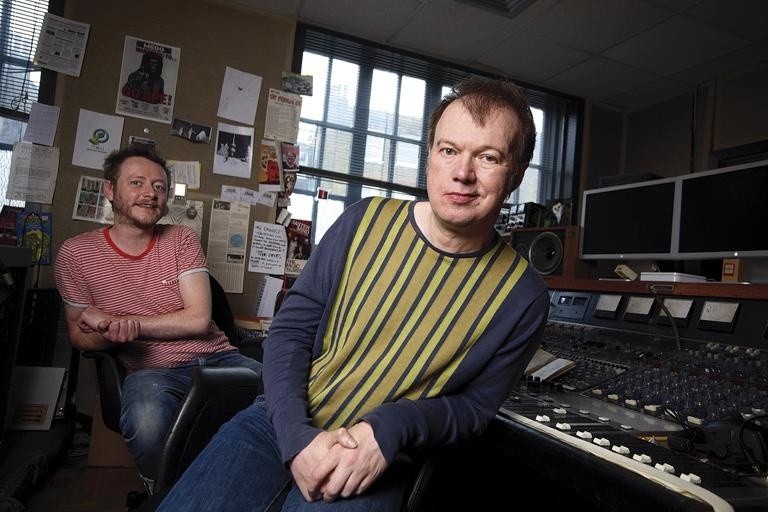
xmin=524 ymin=347 xmax=577 ymax=382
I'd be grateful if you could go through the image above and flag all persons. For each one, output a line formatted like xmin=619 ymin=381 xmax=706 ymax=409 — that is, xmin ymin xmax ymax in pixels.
xmin=53 ymin=145 xmax=262 ymax=495
xmin=156 ymin=77 xmax=551 ymax=511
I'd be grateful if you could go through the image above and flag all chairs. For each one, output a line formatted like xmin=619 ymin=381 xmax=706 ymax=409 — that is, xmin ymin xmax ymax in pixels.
xmin=81 ymin=272 xmax=241 ymax=511
xmin=152 ymin=361 xmax=439 ymax=512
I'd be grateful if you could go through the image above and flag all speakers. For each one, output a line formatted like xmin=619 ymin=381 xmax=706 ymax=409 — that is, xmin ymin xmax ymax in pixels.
xmin=508 ymin=225 xmax=581 ymax=279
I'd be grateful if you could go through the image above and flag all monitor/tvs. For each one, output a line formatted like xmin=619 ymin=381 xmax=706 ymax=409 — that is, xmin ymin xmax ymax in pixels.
xmin=674 ymin=158 xmax=768 ymax=259
xmin=579 ymin=176 xmax=675 ymax=266
